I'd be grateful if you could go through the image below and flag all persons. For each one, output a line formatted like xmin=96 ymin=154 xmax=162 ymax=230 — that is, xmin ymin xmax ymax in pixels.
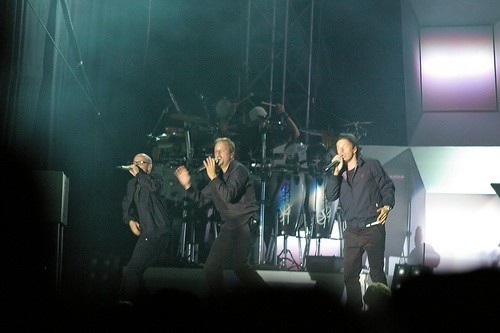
xmin=324 ymin=133 xmax=396 ymax=310
xmin=174 ymin=138 xmax=264 ymax=284
xmin=364 ymin=283 xmax=391 ymax=310
xmin=121 ymin=153 xmax=172 ymax=281
xmin=221 ymin=101 xmax=300 ymax=163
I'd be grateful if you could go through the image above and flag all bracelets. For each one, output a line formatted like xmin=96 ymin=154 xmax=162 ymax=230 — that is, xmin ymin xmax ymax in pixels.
xmin=281 ymin=112 xmax=288 ymax=120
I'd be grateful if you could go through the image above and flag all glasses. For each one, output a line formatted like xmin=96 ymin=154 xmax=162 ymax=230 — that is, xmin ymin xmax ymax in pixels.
xmin=134 ymin=161 xmax=149 ymax=164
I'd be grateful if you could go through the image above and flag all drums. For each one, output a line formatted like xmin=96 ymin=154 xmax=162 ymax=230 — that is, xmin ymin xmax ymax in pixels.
xmin=266 ymin=167 xmax=306 ymax=236
xmin=304 ymin=171 xmax=339 ymax=236
xmin=258 ymin=120 xmax=289 ymax=142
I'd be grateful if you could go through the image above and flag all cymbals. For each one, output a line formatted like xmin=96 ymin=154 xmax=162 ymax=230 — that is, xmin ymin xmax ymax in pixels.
xmin=169 ymin=113 xmax=210 ymax=125
xmin=341 ymin=122 xmax=375 ymax=127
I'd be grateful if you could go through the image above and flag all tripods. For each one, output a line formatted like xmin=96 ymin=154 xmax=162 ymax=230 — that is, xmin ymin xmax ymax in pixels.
xmin=277 ymin=217 xmax=300 ymax=271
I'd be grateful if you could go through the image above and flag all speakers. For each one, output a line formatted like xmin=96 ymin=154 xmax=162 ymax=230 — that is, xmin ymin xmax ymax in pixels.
xmin=29 ymin=170 xmax=69 ymax=226
xmin=390 ymin=264 xmax=430 ymax=291
xmin=144 ymin=266 xmax=208 ymax=305
xmin=306 ymin=255 xmax=344 ymax=275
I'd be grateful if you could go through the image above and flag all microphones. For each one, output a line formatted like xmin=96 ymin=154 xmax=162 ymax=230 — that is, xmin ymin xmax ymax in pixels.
xmin=324 ymin=157 xmax=343 ymax=171
xmin=121 ymin=166 xmax=133 ymax=170
xmin=197 ymin=159 xmax=218 ymax=172
